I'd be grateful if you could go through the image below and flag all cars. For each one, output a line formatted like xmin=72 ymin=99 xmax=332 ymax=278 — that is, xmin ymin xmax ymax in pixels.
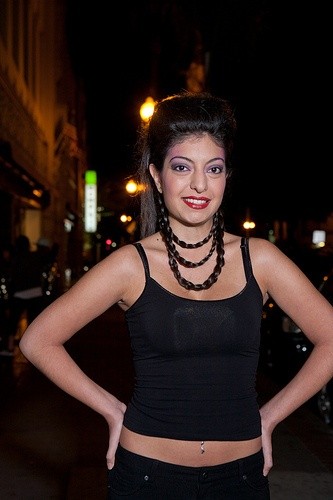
xmin=252 ymin=243 xmax=332 ymax=424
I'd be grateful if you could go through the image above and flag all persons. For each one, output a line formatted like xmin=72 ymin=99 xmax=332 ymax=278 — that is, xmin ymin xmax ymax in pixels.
xmin=19 ymin=95 xmax=333 ymax=499
